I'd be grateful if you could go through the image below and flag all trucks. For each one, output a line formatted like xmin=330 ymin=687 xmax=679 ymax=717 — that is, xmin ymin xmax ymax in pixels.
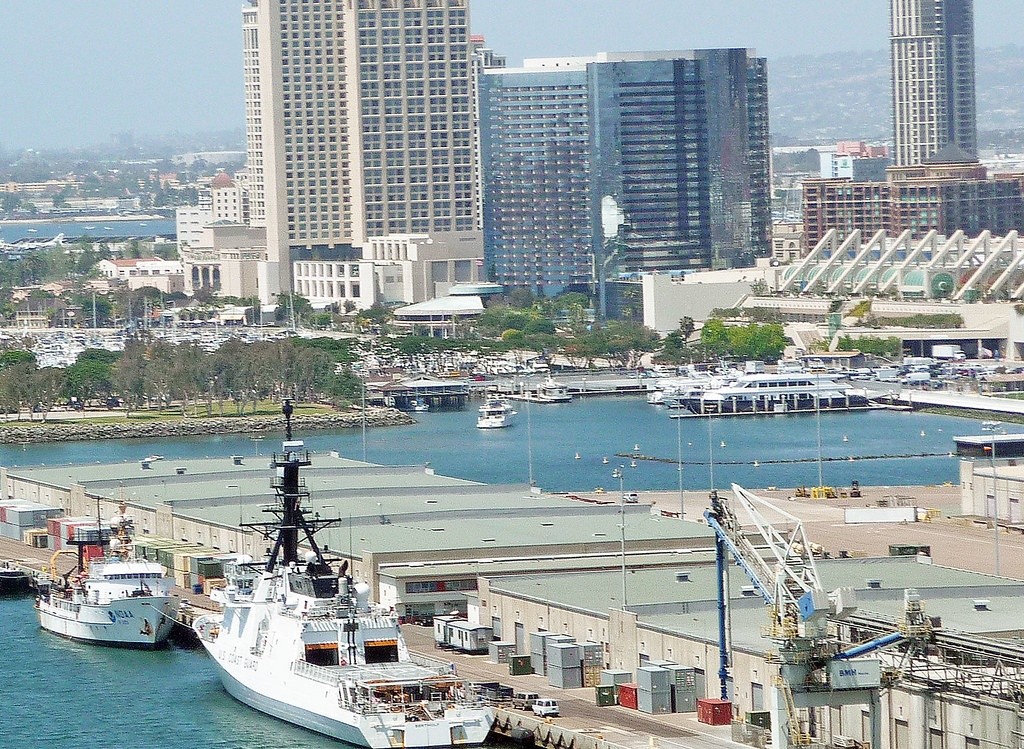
xmin=743 ymin=360 xmax=764 ymax=375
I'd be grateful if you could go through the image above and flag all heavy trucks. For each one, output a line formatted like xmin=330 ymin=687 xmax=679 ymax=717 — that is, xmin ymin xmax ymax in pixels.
xmin=931 ymin=345 xmax=966 ymax=361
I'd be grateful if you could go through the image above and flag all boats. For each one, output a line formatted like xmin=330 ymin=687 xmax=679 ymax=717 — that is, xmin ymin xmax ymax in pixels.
xmin=540 ymin=375 xmax=574 ymax=402
xmin=0 ymin=561 xmax=29 ymax=587
xmin=628 ymin=371 xmax=871 ymax=414
xmin=32 ymin=478 xmax=181 ymax=649
xmin=476 ymin=395 xmax=518 ymax=428
xmin=190 ymin=399 xmax=498 ymax=749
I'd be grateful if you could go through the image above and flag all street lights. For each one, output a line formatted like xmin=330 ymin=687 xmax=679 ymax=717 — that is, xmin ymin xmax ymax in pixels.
xmin=984 ymin=420 xmax=1001 ymax=575
xmin=613 ymin=470 xmax=628 ymax=611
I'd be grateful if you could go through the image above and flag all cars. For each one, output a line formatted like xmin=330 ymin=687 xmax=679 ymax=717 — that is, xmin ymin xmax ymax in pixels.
xmin=812 ymin=365 xmax=1024 ymax=387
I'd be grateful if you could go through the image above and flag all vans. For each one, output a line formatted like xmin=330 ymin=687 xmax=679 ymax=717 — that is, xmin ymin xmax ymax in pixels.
xmin=531 ymin=698 xmax=560 ymax=717
xmin=512 ymin=691 xmax=539 ymax=711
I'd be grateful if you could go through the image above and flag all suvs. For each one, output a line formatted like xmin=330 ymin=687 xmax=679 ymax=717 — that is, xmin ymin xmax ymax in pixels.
xmin=415 ymin=616 xmax=434 ymax=626
xmin=623 ymin=493 xmax=638 ymax=503
